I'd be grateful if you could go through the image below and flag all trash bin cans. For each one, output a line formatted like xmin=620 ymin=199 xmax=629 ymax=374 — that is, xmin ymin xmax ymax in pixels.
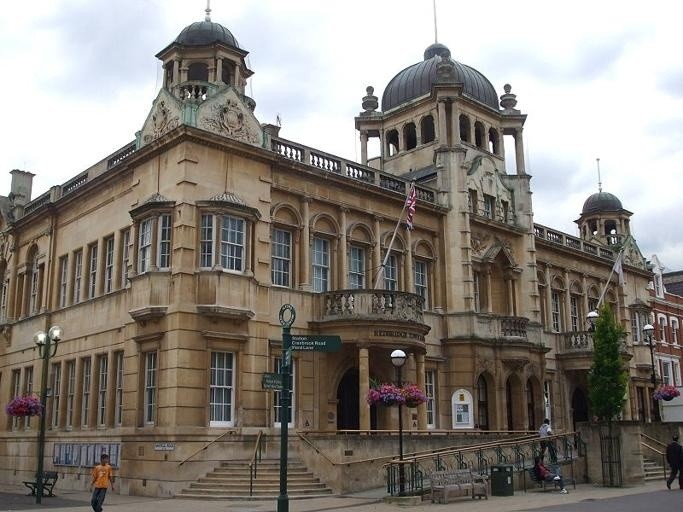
xmin=491 ymin=465 xmax=514 ymax=496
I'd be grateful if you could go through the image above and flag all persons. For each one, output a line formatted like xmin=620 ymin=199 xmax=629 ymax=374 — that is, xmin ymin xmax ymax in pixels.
xmin=533 ymin=456 xmax=568 ymax=495
xmin=539 ymin=418 xmax=557 ymax=463
xmin=90 ymin=454 xmax=114 ymax=512
xmin=666 ymin=434 xmax=683 ymax=489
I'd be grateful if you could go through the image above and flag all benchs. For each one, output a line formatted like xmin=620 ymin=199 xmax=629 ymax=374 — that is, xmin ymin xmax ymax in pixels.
xmin=428 ymin=468 xmax=489 ymax=504
xmin=22 ymin=470 xmax=59 ymax=497
xmin=526 ymin=463 xmax=576 ymax=493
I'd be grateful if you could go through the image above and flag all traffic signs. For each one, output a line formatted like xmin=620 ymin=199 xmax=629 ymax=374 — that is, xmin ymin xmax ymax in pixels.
xmin=260 ymin=372 xmax=285 ymax=393
xmin=284 ymin=333 xmax=344 ymax=355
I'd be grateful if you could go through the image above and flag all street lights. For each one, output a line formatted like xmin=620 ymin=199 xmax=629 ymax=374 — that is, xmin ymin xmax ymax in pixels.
xmin=582 ymin=309 xmax=602 ymax=356
xmin=389 ymin=348 xmax=410 ymax=498
xmin=641 ymin=322 xmax=662 ymax=422
xmin=33 ymin=323 xmax=65 ymax=505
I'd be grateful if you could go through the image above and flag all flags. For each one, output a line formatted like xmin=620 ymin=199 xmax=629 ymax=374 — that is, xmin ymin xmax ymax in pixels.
xmin=613 ymin=254 xmax=626 ymax=287
xmin=405 ymin=182 xmax=416 ymax=233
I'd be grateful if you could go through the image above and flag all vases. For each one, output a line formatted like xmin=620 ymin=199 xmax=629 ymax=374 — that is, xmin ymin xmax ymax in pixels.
xmin=662 ymin=395 xmax=673 ymax=401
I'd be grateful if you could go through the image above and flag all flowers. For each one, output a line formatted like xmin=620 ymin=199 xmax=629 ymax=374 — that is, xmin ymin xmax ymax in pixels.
xmin=4 ymin=393 xmax=45 ymax=417
xmin=365 ymin=382 xmax=434 ymax=408
xmin=651 ymin=382 xmax=681 ymax=401
